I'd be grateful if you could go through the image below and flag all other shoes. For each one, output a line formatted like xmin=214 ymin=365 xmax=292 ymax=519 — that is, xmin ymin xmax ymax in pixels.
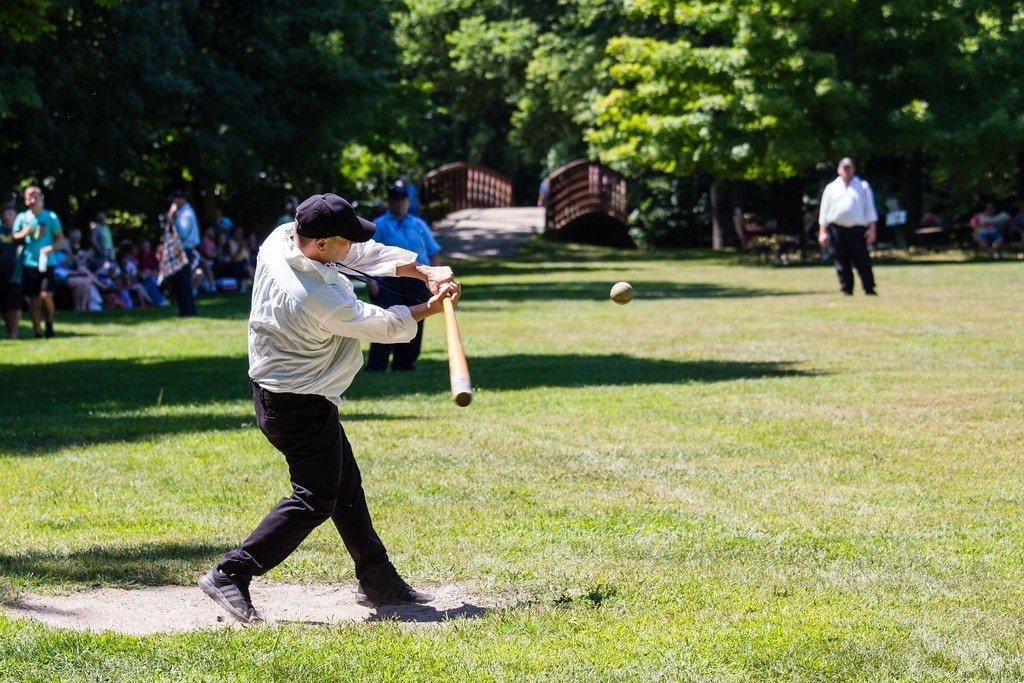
xmin=35 ymin=329 xmax=44 ymax=336
xmin=46 ymin=329 xmax=57 ymax=338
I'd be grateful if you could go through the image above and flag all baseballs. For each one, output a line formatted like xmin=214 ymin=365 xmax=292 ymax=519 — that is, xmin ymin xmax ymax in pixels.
xmin=610 ymin=281 xmax=633 ymax=305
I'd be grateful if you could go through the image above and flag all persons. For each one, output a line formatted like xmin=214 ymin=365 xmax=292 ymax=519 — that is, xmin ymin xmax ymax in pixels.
xmin=364 ymin=180 xmax=445 ymax=373
xmin=400 ymin=174 xmax=421 ymax=216
xmin=197 ymin=193 xmax=462 ymax=628
xmin=538 ymin=175 xmax=551 ymax=206
xmin=740 ymin=208 xmax=790 ymax=265
xmin=969 ymin=203 xmax=1011 ymax=259
xmin=0 ymin=177 xmax=294 ymax=339
xmin=817 ymin=158 xmax=879 ymax=296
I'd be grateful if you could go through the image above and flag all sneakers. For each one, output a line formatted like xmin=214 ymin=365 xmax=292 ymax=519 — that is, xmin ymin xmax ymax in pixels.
xmin=198 ymin=565 xmax=262 ymax=625
xmin=356 ymin=569 xmax=436 ymax=606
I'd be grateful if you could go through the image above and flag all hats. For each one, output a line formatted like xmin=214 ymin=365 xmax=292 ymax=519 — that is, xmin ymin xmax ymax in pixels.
xmin=386 ymin=181 xmax=409 ymax=198
xmin=838 ymin=158 xmax=853 ymax=169
xmin=294 ymin=194 xmax=377 ymax=242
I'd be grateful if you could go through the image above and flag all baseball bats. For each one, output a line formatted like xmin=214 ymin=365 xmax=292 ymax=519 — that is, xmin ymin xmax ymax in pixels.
xmin=440 ymin=282 xmax=473 ymax=407
